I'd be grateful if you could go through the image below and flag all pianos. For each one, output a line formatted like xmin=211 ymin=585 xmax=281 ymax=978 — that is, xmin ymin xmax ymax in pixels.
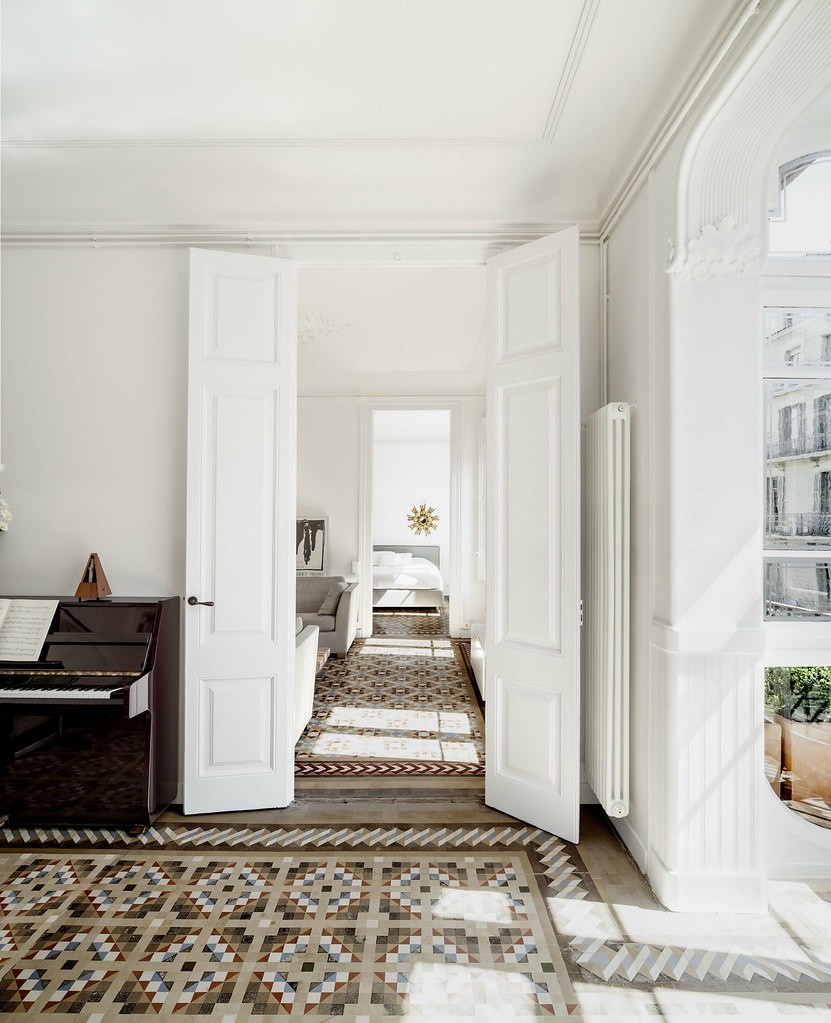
xmin=0 ymin=594 xmax=180 ymax=832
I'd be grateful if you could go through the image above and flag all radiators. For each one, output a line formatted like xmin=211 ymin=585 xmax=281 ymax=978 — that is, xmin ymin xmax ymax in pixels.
xmin=582 ymin=400 xmax=634 ymax=818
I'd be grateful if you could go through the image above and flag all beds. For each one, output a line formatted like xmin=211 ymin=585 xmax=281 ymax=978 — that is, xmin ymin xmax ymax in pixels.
xmin=371 ymin=546 xmax=443 ymax=614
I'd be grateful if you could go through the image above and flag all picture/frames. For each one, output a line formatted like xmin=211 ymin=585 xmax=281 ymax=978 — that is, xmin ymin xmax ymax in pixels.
xmin=296 ymin=517 xmax=327 ymax=571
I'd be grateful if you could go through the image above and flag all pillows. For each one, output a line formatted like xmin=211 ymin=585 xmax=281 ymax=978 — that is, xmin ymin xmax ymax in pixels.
xmin=296 ymin=616 xmax=304 ymax=634
xmin=371 ymin=551 xmax=393 ymax=566
xmin=378 ymin=552 xmax=413 ymax=568
xmin=316 ymin=580 xmax=349 ymax=615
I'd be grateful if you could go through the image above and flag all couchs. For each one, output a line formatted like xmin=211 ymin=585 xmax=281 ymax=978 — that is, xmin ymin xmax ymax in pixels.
xmin=296 ymin=575 xmax=359 ymax=659
xmin=295 ymin=617 xmax=319 ymax=744
